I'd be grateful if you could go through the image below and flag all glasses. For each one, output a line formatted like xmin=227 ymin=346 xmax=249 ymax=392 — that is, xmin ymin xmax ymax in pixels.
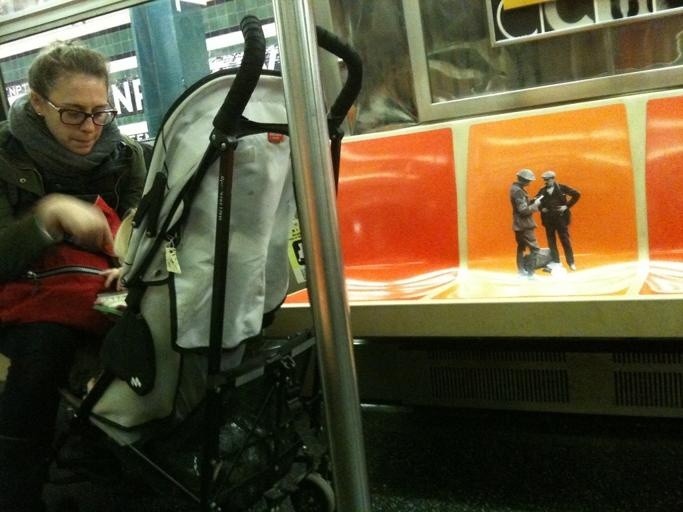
xmin=43 ymin=98 xmax=116 ymax=127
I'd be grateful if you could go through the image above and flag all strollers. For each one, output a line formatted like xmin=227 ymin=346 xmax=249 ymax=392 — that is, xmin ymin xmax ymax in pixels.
xmin=24 ymin=16 xmax=364 ymax=511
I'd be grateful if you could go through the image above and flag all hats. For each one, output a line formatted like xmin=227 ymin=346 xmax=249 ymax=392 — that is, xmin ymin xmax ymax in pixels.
xmin=515 ymin=168 xmax=536 ymax=182
xmin=542 ymin=171 xmax=555 ymax=178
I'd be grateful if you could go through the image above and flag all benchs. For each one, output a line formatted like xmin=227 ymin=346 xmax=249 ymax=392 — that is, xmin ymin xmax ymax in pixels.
xmin=263 ymin=89 xmax=683 ymax=342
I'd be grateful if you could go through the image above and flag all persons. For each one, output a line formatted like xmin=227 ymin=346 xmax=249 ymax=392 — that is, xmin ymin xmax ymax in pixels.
xmin=99 ymin=264 xmax=126 ymax=293
xmin=507 ymin=169 xmax=543 ymax=276
xmin=0 ymin=44 xmax=162 ymax=509
xmin=527 ymin=170 xmax=581 ymax=271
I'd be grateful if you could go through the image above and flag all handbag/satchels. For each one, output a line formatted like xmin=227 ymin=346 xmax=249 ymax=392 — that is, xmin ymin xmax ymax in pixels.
xmin=0 ymin=193 xmax=124 ymax=330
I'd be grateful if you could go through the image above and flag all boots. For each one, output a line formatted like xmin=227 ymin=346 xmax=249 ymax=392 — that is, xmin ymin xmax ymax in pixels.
xmin=0 ymin=435 xmax=48 ymax=511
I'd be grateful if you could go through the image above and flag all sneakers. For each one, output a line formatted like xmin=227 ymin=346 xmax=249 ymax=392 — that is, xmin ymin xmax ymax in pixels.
xmin=517 ymin=262 xmax=577 ymax=280
